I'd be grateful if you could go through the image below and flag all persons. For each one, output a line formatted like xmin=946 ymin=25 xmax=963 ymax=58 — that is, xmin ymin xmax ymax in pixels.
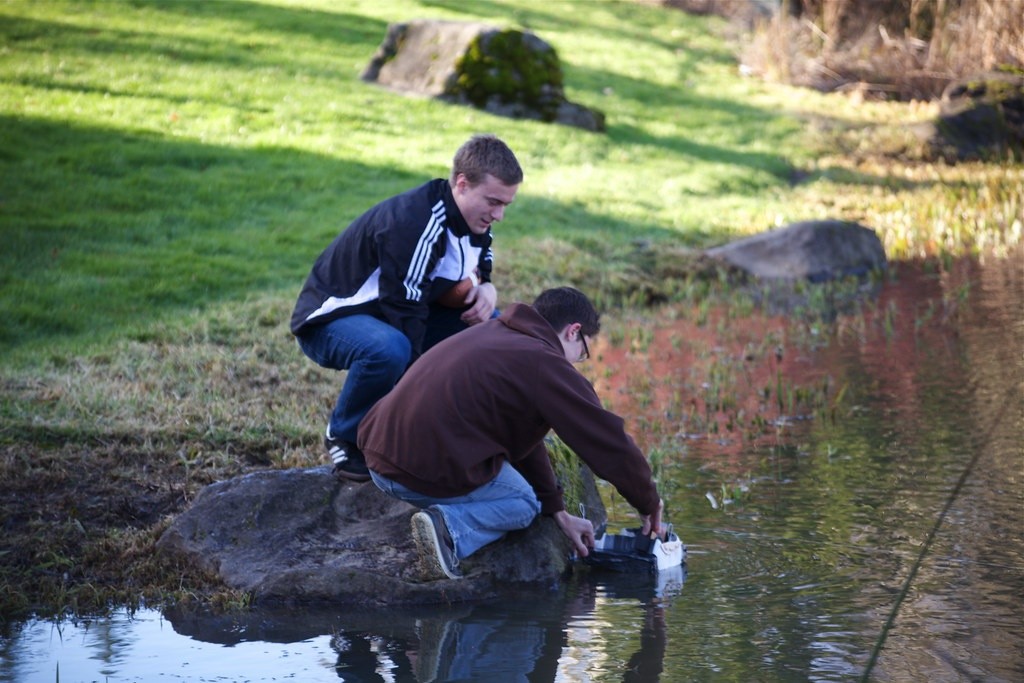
xmin=355 ymin=287 xmax=665 ymax=580
xmin=291 ymin=135 xmax=523 ymax=482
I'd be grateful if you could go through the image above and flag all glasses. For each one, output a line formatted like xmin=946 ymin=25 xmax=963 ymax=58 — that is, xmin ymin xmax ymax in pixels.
xmin=576 ymin=328 xmax=591 ymax=365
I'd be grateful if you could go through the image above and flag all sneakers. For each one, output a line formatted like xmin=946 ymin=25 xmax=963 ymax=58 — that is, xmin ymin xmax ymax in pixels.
xmin=412 ymin=504 xmax=466 ymax=581
xmin=323 ymin=423 xmax=373 ymax=481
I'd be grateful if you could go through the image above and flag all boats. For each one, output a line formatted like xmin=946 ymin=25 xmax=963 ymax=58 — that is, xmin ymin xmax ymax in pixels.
xmin=586 ymin=552 xmax=687 ymax=607
xmin=578 ymin=522 xmax=687 ymax=572
